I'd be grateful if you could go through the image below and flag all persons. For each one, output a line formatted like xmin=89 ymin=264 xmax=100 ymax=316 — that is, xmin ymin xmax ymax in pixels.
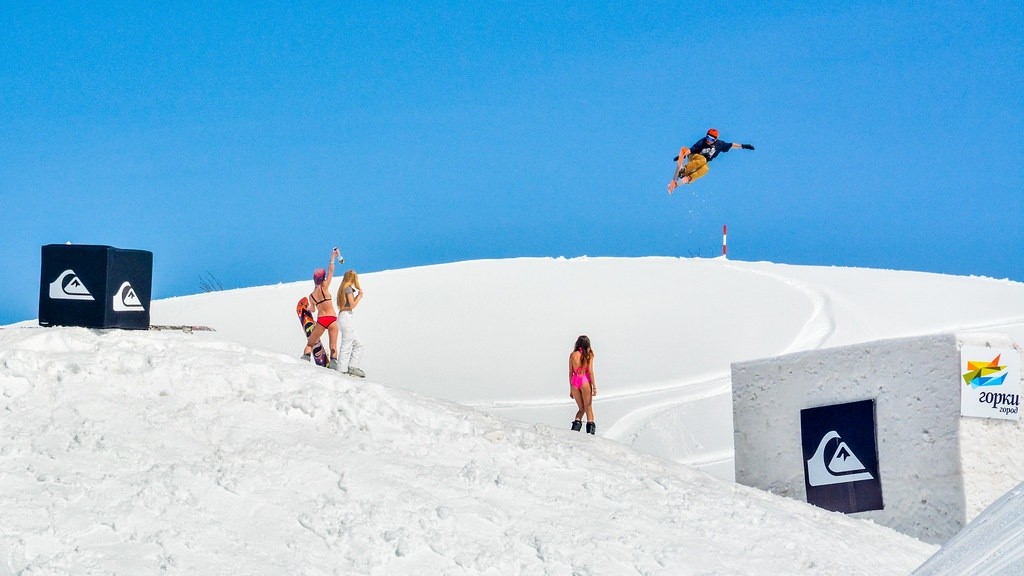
xmin=673 ymin=128 xmax=756 ymax=197
xmin=301 ymin=247 xmax=340 ymax=369
xmin=568 ymin=334 xmax=597 ymax=435
xmin=337 ymin=269 xmax=366 ymax=378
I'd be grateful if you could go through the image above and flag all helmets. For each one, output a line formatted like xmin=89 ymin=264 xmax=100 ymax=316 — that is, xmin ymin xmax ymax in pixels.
xmin=707 ymin=129 xmax=718 ymax=139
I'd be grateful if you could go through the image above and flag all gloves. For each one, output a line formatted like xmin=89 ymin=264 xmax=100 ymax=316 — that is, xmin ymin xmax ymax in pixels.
xmin=674 ymin=155 xmax=686 ymax=161
xmin=742 ymin=144 xmax=754 ymax=150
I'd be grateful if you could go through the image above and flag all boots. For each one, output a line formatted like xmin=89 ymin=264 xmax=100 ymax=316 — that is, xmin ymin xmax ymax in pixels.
xmin=587 ymin=422 xmax=595 ymax=435
xmin=571 ymin=420 xmax=582 ymax=431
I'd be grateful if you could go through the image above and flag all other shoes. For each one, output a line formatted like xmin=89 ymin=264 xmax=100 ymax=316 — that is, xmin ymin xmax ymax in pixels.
xmin=301 ymin=354 xmax=310 ymax=361
xmin=329 ymin=357 xmax=337 ymax=370
xmin=680 ymin=165 xmax=685 ymax=172
xmin=348 ymin=366 xmax=365 ymax=378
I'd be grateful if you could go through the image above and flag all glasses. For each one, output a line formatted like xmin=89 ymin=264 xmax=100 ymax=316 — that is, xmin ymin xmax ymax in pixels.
xmin=706 ymin=135 xmax=718 ymax=143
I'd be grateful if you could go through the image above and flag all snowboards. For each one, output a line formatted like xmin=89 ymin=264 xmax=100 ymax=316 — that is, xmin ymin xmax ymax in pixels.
xmin=667 ymin=145 xmax=687 ymax=197
xmin=294 ymin=296 xmax=330 ymax=368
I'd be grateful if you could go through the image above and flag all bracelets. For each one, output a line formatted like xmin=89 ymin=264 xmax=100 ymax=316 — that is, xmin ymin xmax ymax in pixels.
xmin=593 ymin=387 xmax=596 ymax=389
